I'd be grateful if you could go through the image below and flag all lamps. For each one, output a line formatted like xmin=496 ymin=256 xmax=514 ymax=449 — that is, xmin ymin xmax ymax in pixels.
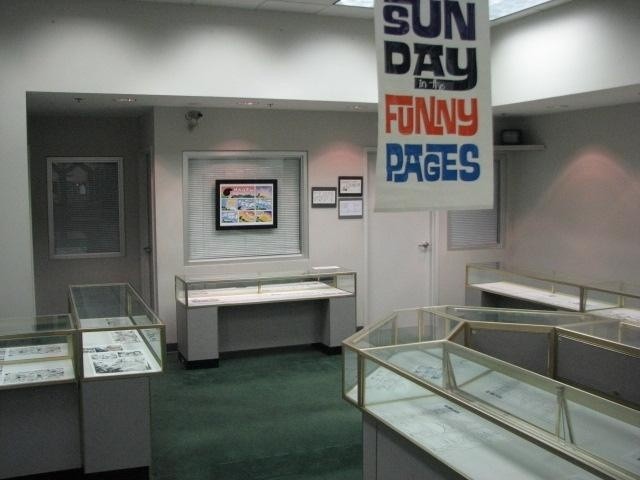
xmin=500 ymin=128 xmax=523 ymax=145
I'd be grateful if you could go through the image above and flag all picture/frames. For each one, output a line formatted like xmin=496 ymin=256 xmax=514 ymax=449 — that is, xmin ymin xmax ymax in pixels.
xmin=215 ymin=178 xmax=278 ymax=230
